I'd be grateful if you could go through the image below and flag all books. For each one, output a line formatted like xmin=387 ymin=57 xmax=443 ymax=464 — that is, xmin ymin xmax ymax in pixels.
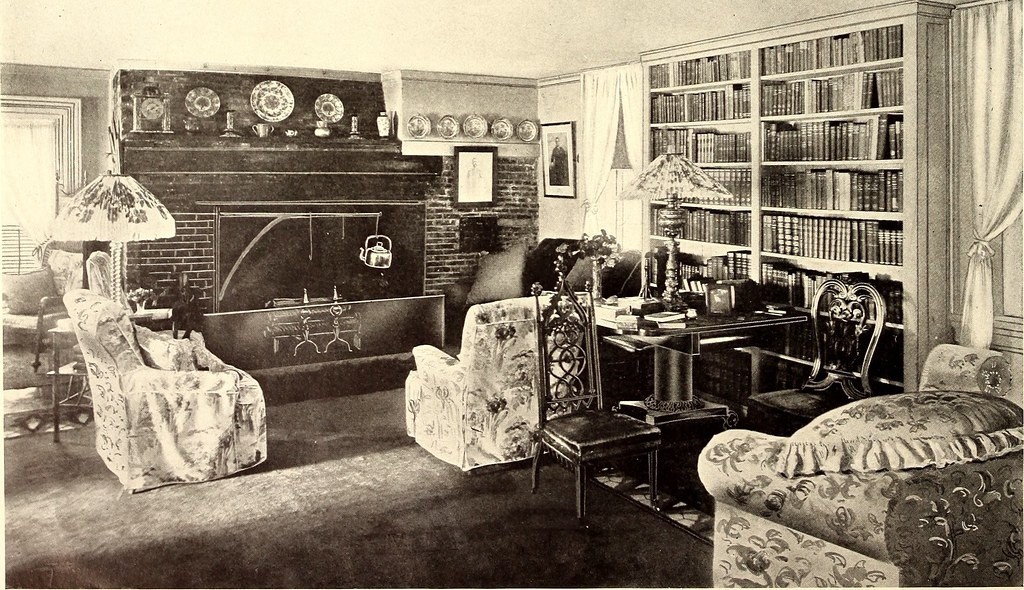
xmin=758 ymin=25 xmax=903 ymax=76
xmin=759 ymin=168 xmax=904 ymax=212
xmin=593 ymin=296 xmax=688 ymax=331
xmin=650 ymin=167 xmax=752 ymax=206
xmin=762 ymin=215 xmax=903 ymax=267
xmin=648 ymin=50 xmax=752 ymax=88
xmin=760 ymin=263 xmax=904 ymax=327
xmin=648 ymin=311 xmax=834 ymax=415
xmin=650 ymin=208 xmax=751 ymax=249
xmin=760 ymin=67 xmax=904 ymax=117
xmin=650 ymin=128 xmax=751 ymax=164
xmin=651 ymin=251 xmax=751 ymax=291
xmin=761 ymin=115 xmax=904 ymax=161
xmin=650 ymin=82 xmax=752 ymax=124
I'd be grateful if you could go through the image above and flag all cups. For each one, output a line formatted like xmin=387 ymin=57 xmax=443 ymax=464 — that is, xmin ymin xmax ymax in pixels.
xmin=250 ymin=123 xmax=269 ymax=139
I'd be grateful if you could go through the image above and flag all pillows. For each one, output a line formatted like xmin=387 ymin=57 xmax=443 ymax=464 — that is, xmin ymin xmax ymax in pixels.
xmin=2 ymin=266 xmax=58 ymax=316
xmin=777 ymin=390 xmax=1024 ymax=476
xmin=465 ymin=238 xmax=528 ymax=305
xmin=132 ymin=325 xmax=195 ymax=372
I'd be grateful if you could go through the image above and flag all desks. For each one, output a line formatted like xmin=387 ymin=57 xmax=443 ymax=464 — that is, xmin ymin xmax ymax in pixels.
xmin=597 ymin=310 xmax=807 ymax=432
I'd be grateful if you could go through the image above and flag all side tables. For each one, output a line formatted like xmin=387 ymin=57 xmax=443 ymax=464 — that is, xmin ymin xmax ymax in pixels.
xmin=47 ymin=328 xmax=93 ymax=443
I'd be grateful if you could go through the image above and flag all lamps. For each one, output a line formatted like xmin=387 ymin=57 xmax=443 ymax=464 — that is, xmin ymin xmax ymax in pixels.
xmin=618 ymin=152 xmax=733 ymax=310
xmin=49 ymin=171 xmax=175 ymax=304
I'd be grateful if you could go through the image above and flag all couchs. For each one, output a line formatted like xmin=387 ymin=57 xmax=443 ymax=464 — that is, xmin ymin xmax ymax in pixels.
xmin=447 ymin=237 xmax=641 ymax=348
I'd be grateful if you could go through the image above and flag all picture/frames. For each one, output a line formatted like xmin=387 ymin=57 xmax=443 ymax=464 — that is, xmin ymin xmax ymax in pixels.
xmin=454 ymin=146 xmax=498 ymax=206
xmin=539 ymin=121 xmax=577 ymax=198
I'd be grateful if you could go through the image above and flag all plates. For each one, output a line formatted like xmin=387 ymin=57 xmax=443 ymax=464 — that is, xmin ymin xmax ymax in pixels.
xmin=436 ymin=115 xmax=459 ymax=139
xmin=185 ymin=87 xmax=220 ymax=116
xmin=407 ymin=114 xmax=431 ymax=139
xmin=516 ymin=119 xmax=539 ymax=143
xmin=491 ymin=118 xmax=514 ymax=141
xmin=462 ymin=114 xmax=488 ymax=140
xmin=250 ymin=80 xmax=295 ymax=122
xmin=315 ymin=93 xmax=345 ymax=124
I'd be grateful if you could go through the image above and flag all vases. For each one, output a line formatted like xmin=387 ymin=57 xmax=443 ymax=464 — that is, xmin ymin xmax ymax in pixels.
xmin=593 ymin=260 xmax=603 ymax=298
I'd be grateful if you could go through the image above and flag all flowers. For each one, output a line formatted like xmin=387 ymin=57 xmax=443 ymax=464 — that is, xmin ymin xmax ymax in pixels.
xmin=555 ymin=229 xmax=622 ymax=291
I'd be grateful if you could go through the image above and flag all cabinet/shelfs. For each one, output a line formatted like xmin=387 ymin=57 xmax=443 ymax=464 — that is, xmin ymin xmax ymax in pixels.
xmin=644 ymin=14 xmax=948 ymax=394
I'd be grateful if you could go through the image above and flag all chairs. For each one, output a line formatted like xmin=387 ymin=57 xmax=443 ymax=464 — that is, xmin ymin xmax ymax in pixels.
xmin=406 ymin=293 xmax=589 ymax=471
xmin=2 ymin=240 xmax=267 ymax=494
xmin=698 ymin=343 xmax=1024 ymax=588
xmin=530 ymin=266 xmax=663 ymax=521
xmin=747 ymin=279 xmax=887 ymax=436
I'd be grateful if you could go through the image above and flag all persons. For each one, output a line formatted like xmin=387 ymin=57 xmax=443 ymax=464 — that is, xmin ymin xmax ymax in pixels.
xmin=551 ymin=137 xmax=565 ymax=185
xmin=466 ymin=157 xmax=484 ymax=180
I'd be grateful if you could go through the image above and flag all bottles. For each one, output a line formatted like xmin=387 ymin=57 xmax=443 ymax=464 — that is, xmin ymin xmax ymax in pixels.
xmin=377 ymin=111 xmax=391 ymax=137
xmin=315 ymin=120 xmax=330 ymax=137
